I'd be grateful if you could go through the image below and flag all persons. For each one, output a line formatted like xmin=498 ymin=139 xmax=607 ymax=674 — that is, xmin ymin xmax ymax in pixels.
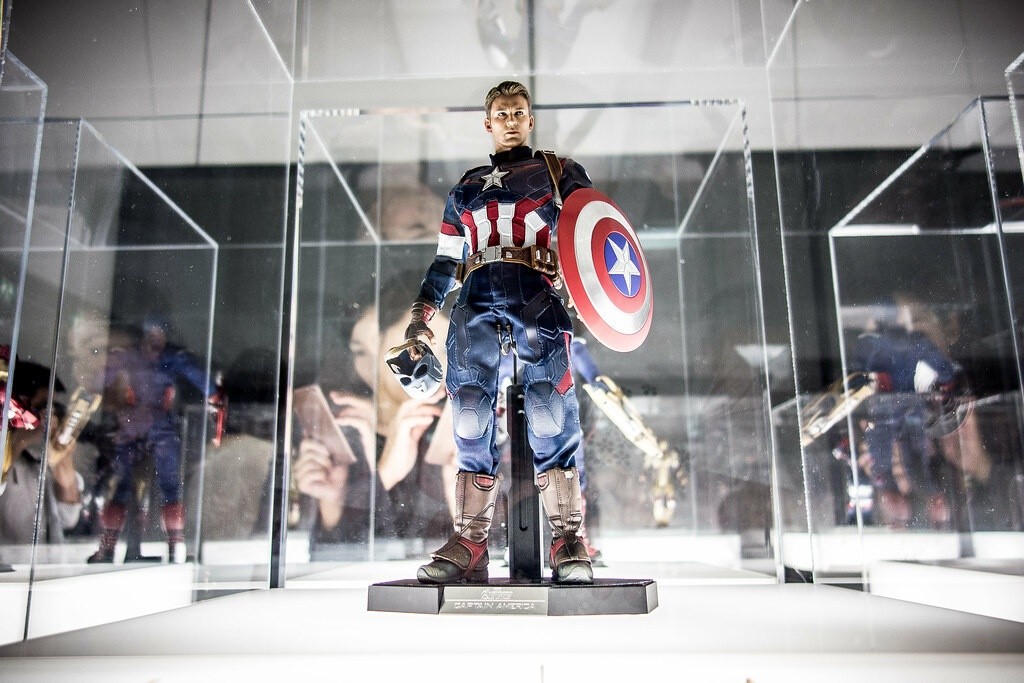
xmin=62 ymin=309 xmax=223 ymax=564
xmin=295 ymin=271 xmax=460 ymax=561
xmin=852 ymin=296 xmax=962 ymax=524
xmin=408 ymin=81 xmax=594 ymax=585
xmin=0 ymin=280 xmax=277 ymax=545
xmin=344 ymin=139 xmax=447 ymax=244
xmin=846 ymin=275 xmax=1024 ymax=528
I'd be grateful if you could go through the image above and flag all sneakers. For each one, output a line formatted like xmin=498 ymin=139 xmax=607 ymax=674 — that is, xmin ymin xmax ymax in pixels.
xmin=416 ymin=538 xmax=488 ymax=584
xmin=549 ymin=535 xmax=595 ymax=584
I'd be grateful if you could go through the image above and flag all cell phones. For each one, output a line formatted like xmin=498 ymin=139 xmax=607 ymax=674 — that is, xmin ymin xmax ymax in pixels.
xmin=295 ymin=385 xmax=357 ymax=468
xmin=424 ymin=398 xmax=457 ymax=465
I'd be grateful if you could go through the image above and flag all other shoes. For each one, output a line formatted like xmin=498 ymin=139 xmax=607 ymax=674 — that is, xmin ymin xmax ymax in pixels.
xmin=87 ymin=549 xmax=115 ymax=562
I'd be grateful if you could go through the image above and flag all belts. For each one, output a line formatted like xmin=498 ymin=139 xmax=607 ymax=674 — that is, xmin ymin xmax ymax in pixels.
xmin=460 ymin=245 xmax=561 ymax=286
